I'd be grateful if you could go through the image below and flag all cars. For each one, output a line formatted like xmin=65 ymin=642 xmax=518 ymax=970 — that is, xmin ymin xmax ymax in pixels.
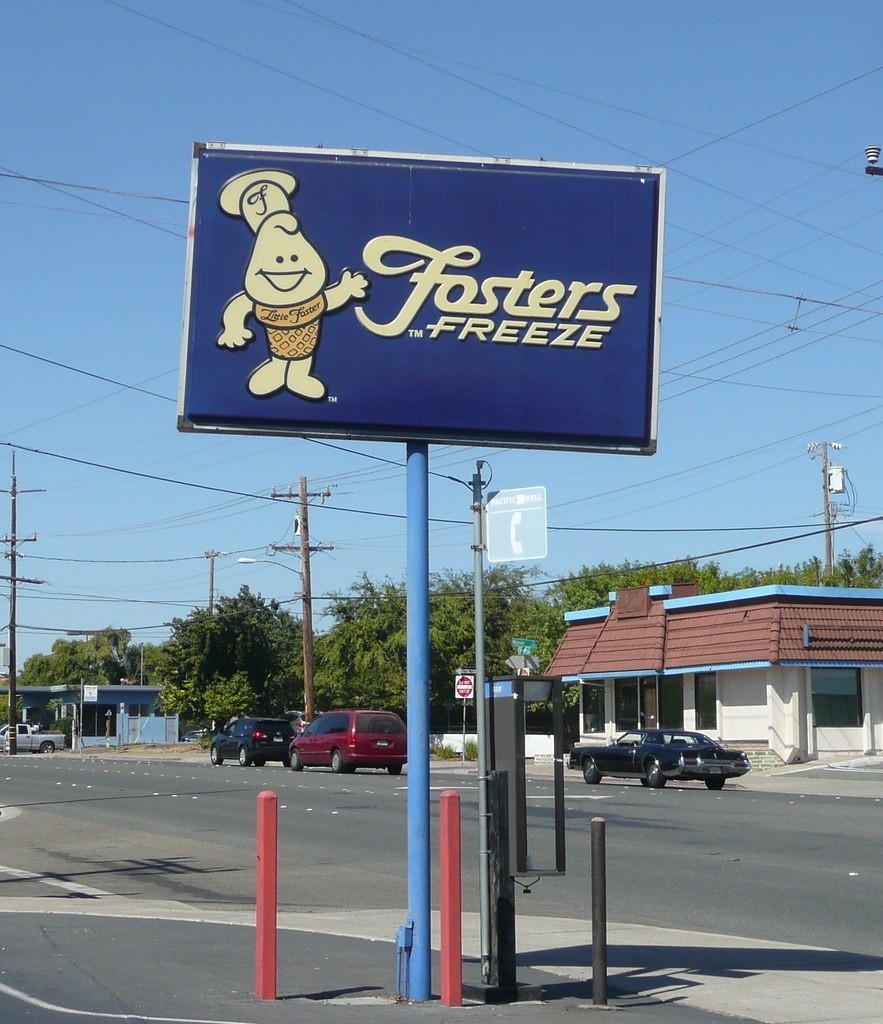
xmin=565 ymin=729 xmax=752 ymax=790
xmin=209 ymin=717 xmax=298 ymax=767
xmin=275 ymin=710 xmax=323 ymax=735
xmin=181 ymin=730 xmax=207 ymax=742
xmin=288 ymin=710 xmax=408 ymax=774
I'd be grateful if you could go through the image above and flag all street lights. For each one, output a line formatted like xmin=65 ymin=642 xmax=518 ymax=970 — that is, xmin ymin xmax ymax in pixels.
xmin=237 ymin=557 xmax=315 ymax=724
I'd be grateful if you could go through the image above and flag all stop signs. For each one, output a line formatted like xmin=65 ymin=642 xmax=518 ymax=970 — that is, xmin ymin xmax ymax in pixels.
xmin=456 ymin=676 xmax=473 ymax=698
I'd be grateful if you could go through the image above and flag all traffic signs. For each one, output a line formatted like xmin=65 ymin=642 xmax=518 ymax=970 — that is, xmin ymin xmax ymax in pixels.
xmin=454 ymin=666 xmax=478 ymax=675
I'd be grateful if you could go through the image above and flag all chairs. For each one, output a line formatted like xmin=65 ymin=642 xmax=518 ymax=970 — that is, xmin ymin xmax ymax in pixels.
xmin=671 ymin=739 xmax=686 ymax=743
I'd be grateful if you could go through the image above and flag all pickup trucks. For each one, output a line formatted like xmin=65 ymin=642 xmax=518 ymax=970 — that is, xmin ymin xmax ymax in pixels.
xmin=0 ymin=724 xmax=66 ymax=754
